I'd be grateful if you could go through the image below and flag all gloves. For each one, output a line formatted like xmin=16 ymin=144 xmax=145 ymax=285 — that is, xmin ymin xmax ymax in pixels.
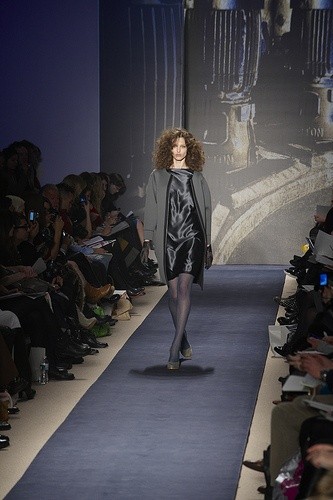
xmin=140 ymin=241 xmax=150 ymax=270
xmin=204 ymin=244 xmax=213 ymax=270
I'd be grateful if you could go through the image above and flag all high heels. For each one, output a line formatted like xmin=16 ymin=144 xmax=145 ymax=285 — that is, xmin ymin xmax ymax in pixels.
xmin=181 ymin=328 xmax=193 ymax=358
xmin=167 ymin=353 xmax=181 ymax=370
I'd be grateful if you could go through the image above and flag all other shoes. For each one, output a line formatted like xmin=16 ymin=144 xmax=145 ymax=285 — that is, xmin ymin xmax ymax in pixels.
xmin=243 ymin=254 xmax=295 ymax=500
xmin=0 ymin=259 xmax=166 ymax=448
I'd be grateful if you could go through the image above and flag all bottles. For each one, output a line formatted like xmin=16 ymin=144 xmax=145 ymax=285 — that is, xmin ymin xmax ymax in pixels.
xmin=39 ymin=355 xmax=49 ymax=385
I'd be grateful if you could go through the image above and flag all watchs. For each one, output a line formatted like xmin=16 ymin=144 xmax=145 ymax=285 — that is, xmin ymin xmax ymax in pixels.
xmin=319 ymin=369 xmax=328 ymax=382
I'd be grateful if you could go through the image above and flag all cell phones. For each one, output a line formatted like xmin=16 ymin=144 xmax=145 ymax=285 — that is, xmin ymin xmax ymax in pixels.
xmin=319 ymin=274 xmax=328 ymax=288
xmin=80 ymin=196 xmax=87 ymax=206
xmin=28 ymin=210 xmax=37 ymax=224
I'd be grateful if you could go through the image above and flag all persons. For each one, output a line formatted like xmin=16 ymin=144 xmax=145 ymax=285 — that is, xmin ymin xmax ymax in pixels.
xmin=140 ymin=128 xmax=214 ymax=369
xmin=0 ymin=142 xmax=157 ymax=449
xmin=242 ymin=201 xmax=333 ymax=500
